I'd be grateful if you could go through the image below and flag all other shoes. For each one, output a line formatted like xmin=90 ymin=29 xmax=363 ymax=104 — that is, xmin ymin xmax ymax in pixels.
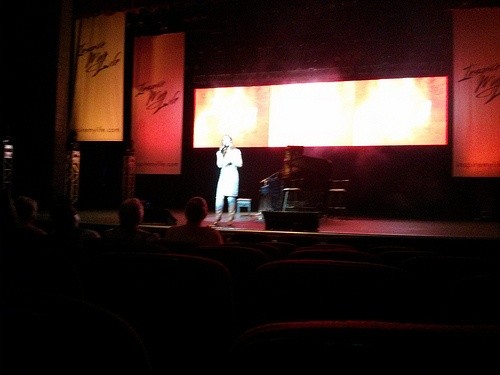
xmin=214 ymin=221 xmax=218 ymax=224
xmin=227 ymin=220 xmax=232 ymax=226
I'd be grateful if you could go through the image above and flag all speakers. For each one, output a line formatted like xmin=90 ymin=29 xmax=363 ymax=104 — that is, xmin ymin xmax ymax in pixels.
xmin=262 ymin=211 xmax=320 ymax=233
xmin=140 ymin=207 xmax=177 ymax=226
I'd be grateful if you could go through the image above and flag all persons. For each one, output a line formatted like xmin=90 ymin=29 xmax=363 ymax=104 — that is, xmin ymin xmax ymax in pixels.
xmin=44 ymin=201 xmax=99 ymax=240
xmin=103 ymin=198 xmax=160 ymax=242
xmin=213 ymin=132 xmax=244 ymax=227
xmin=0 ymin=196 xmax=46 ymax=240
xmin=165 ymin=197 xmax=224 ymax=246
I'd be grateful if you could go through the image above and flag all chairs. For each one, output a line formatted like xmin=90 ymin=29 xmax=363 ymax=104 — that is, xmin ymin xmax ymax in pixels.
xmin=0 ymin=232 xmax=499 ymax=375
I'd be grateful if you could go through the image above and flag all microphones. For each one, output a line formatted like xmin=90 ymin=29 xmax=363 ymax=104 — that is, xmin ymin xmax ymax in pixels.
xmin=221 ymin=145 xmax=227 ymax=152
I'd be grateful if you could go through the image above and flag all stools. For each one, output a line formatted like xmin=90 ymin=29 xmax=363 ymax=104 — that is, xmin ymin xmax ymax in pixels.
xmin=237 ymin=198 xmax=253 ymax=212
xmin=281 ymin=188 xmax=300 ymax=210
